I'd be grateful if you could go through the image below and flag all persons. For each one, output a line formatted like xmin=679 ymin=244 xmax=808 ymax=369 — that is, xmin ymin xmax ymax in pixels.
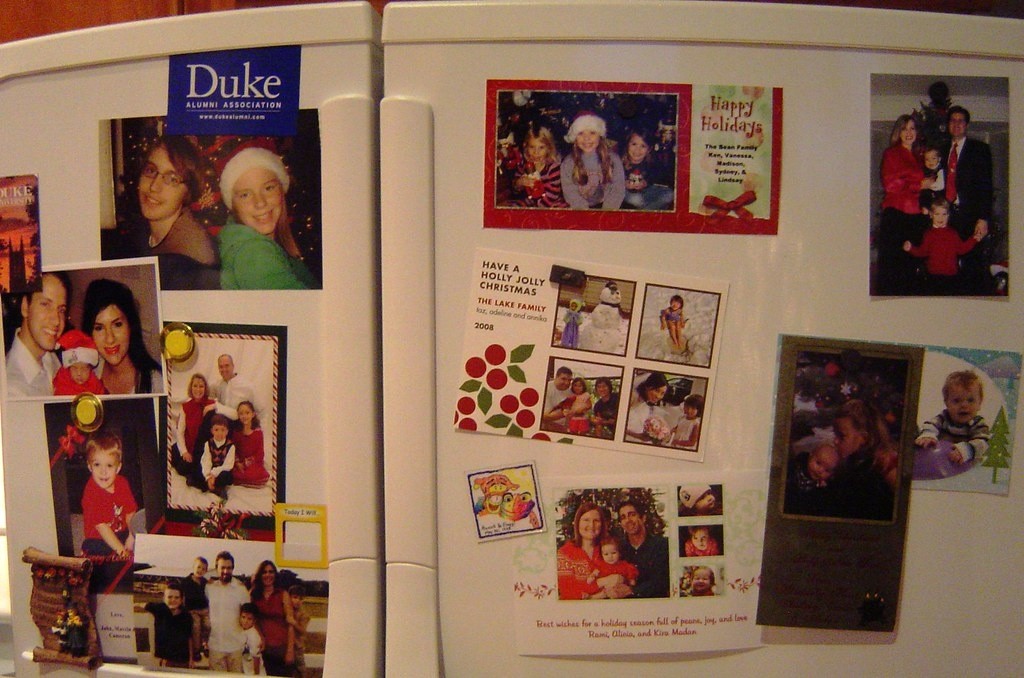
xmin=6 ymin=270 xmax=74 ymax=397
xmin=199 ymin=415 xmax=236 ymax=499
xmin=144 ymin=583 xmax=194 ymax=669
xmin=249 ymin=559 xmax=302 ymax=678
xmin=877 ymin=114 xmax=932 ymax=284
xmin=677 ymin=483 xmax=722 ymax=516
xmin=681 ymin=566 xmax=716 ymax=596
xmin=220 ymin=136 xmax=316 ymax=291
xmin=611 ymin=502 xmax=670 ymax=598
xmin=590 ymin=377 xmax=620 ymax=440
xmin=163 ymin=556 xmax=214 ymax=662
xmin=558 ymin=503 xmax=624 ymax=599
xmin=783 ymin=446 xmax=839 ymax=517
xmin=511 ymin=123 xmax=570 ymax=208
xmin=170 ymin=373 xmax=217 ymax=489
xmin=913 ymin=368 xmax=990 ymax=468
xmin=238 ymin=601 xmax=267 ymax=678
xmin=559 ymin=107 xmax=625 ymax=210
xmin=80 ymin=277 xmax=165 ymax=395
xmin=833 ymin=396 xmax=900 ymax=523
xmin=285 ymin=582 xmax=314 ymax=678
xmin=202 ymin=354 xmax=255 ymax=429
xmin=568 ymin=377 xmax=592 ymax=436
xmin=230 ymin=400 xmax=271 ymax=488
xmin=620 ymin=125 xmax=674 ymax=211
xmin=941 ymin=105 xmax=995 ymax=284
xmin=561 ymin=298 xmax=584 ymax=350
xmin=135 ymin=133 xmax=221 ymax=290
xmin=52 ymin=327 xmax=107 ymax=396
xmin=684 ymin=526 xmax=720 ymax=557
xmin=541 ymin=366 xmax=576 ymax=433
xmin=80 ymin=428 xmax=149 ymax=560
xmin=624 ymin=370 xmax=669 ymax=449
xmin=586 ymin=537 xmax=640 ymax=599
xmin=902 ymin=197 xmax=984 ymax=276
xmin=919 ymin=146 xmax=949 ymax=217
xmin=659 ymin=295 xmax=686 ymax=349
xmin=204 ymin=550 xmax=265 ymax=675
xmin=670 ymin=394 xmax=703 ymax=451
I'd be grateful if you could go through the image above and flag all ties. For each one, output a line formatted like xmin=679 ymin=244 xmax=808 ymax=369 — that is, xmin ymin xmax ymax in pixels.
xmin=944 ymin=142 xmax=959 ymax=204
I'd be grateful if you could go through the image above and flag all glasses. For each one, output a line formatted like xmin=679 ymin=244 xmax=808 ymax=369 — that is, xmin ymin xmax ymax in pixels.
xmin=209 ymin=135 xmax=258 ymax=162
xmin=141 ymin=160 xmax=187 ymax=186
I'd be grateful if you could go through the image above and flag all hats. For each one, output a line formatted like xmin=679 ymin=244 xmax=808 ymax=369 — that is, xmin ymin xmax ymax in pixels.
xmin=50 ymin=330 xmax=101 ymax=370
xmin=679 ymin=484 xmax=712 ymax=509
xmin=207 ymin=131 xmax=290 ymax=210
xmin=564 ymin=110 xmax=608 ymax=143
